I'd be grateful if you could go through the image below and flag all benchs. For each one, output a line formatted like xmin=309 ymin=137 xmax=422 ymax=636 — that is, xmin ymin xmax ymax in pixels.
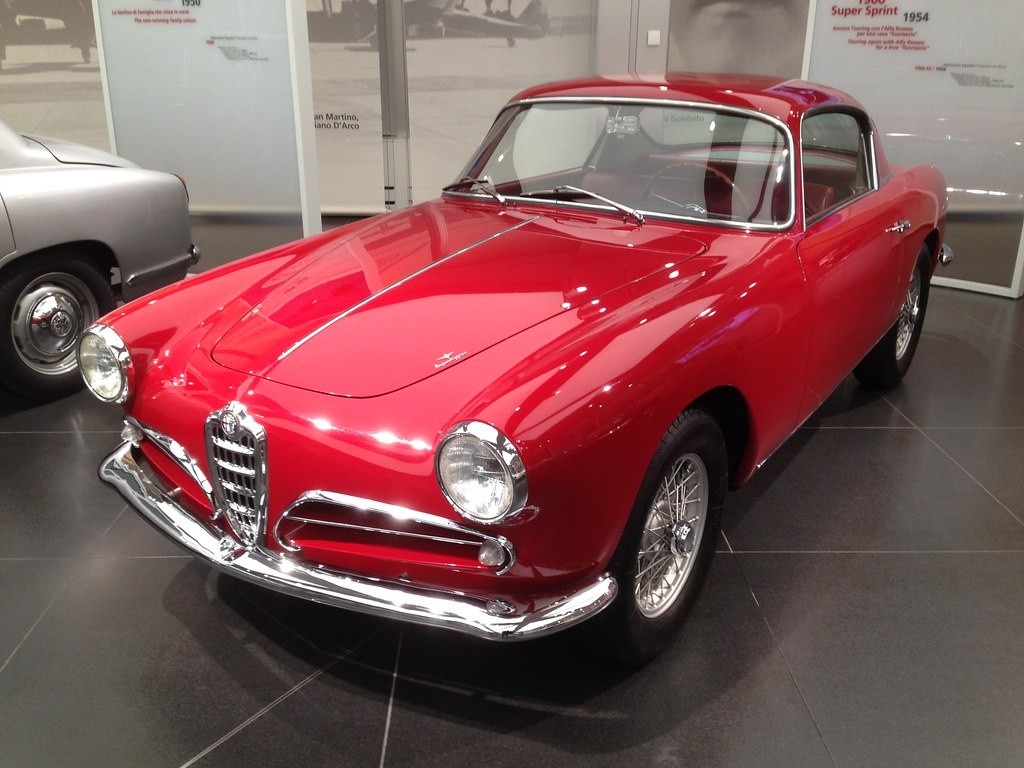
xmin=578 ymin=164 xmax=836 ymax=222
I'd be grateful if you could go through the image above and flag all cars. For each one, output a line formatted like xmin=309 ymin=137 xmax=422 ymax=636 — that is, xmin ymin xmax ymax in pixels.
xmin=76 ymin=69 xmax=955 ymax=644
xmin=1 ymin=114 xmax=203 ymax=403
